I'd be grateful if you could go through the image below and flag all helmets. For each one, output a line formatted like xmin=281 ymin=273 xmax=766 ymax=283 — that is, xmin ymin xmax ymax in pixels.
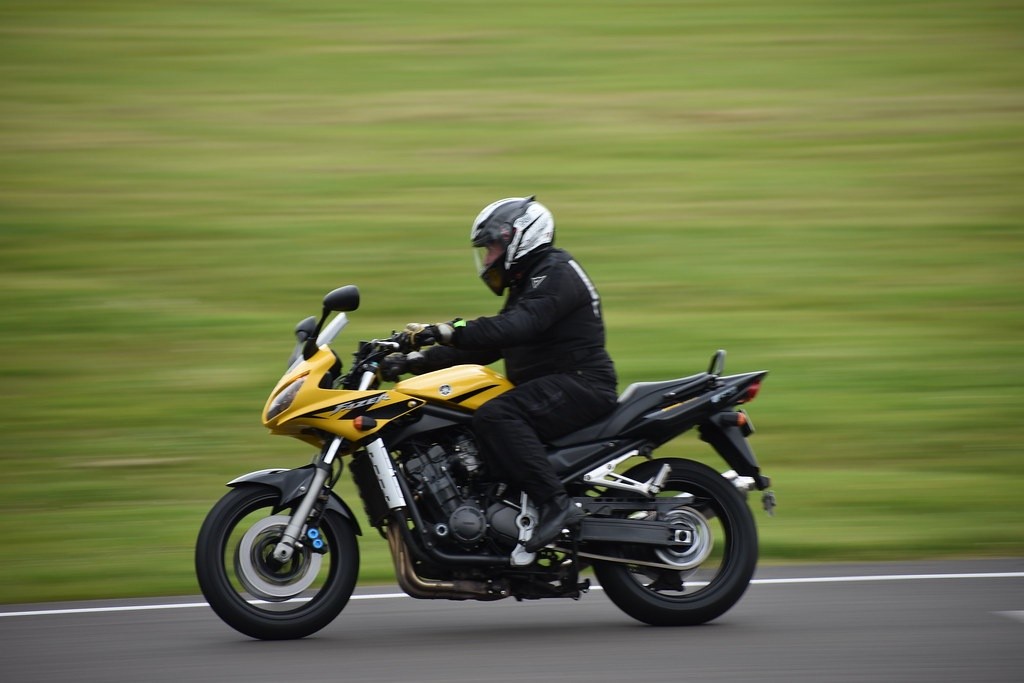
xmin=470 ymin=195 xmax=555 ymax=295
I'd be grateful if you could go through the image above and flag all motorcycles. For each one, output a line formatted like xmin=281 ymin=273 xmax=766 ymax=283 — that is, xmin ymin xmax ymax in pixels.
xmin=193 ymin=284 xmax=768 ymax=639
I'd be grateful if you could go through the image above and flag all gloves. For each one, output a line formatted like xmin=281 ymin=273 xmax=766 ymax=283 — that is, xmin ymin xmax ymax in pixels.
xmin=393 ymin=322 xmax=456 ymax=355
xmin=384 ymin=351 xmax=430 ymax=376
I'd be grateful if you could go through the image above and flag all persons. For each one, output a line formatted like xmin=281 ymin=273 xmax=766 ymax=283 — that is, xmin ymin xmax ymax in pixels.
xmin=378 ymin=193 xmax=618 ymax=553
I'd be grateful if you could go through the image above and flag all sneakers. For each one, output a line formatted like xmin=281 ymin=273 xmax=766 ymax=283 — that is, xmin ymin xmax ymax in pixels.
xmin=526 ymin=497 xmax=586 ymax=553
